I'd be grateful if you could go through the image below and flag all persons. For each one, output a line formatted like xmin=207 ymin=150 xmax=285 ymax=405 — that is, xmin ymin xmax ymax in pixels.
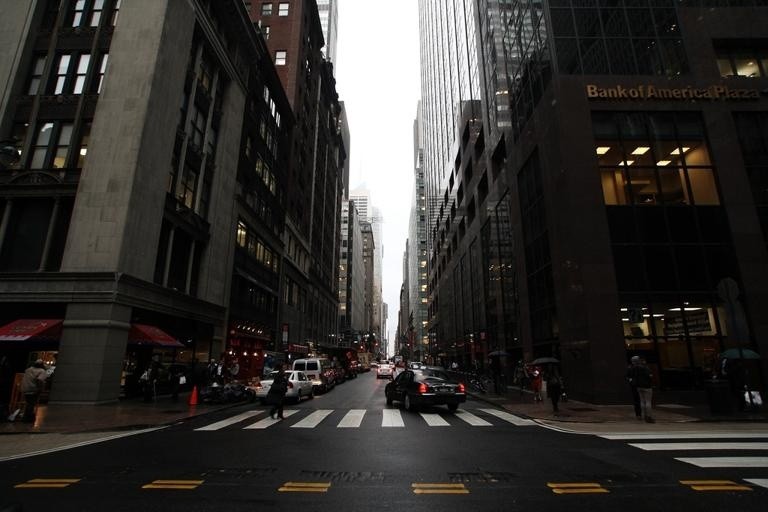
xmin=624 ymin=355 xmax=656 ymax=423
xmin=544 ymin=363 xmax=565 ymax=413
xmin=267 ymin=368 xmax=292 ymax=419
xmin=169 ymin=358 xmax=240 ymax=400
xmin=732 ymin=361 xmax=752 ymax=412
xmin=21 ymin=359 xmax=47 ymax=423
xmin=405 ymin=361 xmax=411 ymax=371
xmin=448 ymin=359 xmax=543 ymax=401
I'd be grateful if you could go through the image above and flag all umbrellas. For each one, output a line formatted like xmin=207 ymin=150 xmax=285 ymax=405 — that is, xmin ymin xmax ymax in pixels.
xmin=487 ymin=351 xmax=507 ymax=360
xmin=720 ymin=347 xmax=762 ymax=360
xmin=532 ymin=357 xmax=560 ymax=365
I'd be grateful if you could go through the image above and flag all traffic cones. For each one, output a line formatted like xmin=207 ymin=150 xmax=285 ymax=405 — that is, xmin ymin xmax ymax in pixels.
xmin=188 ymin=384 xmax=199 ymax=405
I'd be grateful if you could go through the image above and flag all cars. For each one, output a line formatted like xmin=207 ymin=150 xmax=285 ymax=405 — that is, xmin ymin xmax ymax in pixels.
xmin=246 ymin=355 xmax=466 ymax=412
xmin=159 ymin=362 xmax=184 ymax=394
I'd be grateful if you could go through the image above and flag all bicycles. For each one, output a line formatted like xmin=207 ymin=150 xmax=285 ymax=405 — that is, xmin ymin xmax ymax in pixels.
xmin=468 ymin=358 xmax=493 ymax=393
